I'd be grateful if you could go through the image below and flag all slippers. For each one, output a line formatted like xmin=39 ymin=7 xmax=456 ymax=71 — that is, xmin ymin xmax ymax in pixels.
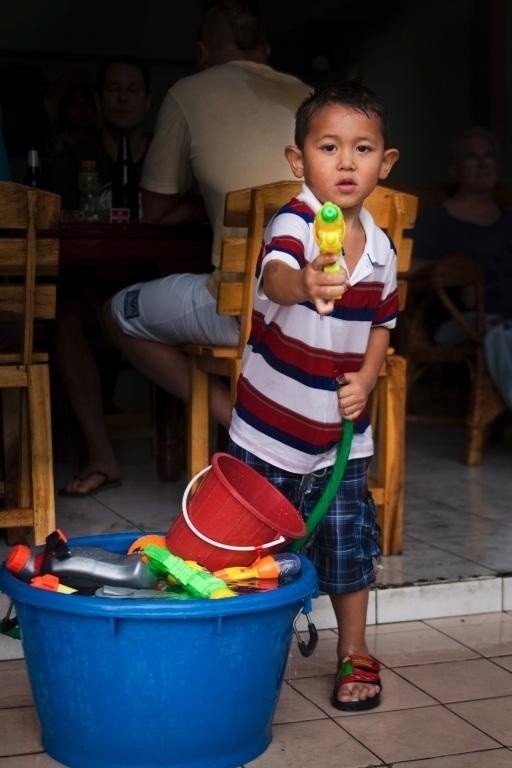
xmin=57 ymin=467 xmax=124 ymax=499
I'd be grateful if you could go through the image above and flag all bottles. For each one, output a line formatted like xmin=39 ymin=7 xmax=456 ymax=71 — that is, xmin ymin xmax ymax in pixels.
xmin=111 ymin=136 xmax=135 ymax=212
xmin=154 ymin=413 xmax=183 ymax=483
xmin=77 ymin=159 xmax=102 ymax=216
xmin=22 ymin=145 xmax=44 ymax=191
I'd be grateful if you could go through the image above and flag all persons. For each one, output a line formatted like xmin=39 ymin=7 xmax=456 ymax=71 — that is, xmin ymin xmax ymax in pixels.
xmin=24 ymin=55 xmax=160 ymax=498
xmin=224 ymin=80 xmax=400 ymax=711
xmin=403 ymin=125 xmax=512 ymax=410
xmin=97 ymin=0 xmax=316 ymax=430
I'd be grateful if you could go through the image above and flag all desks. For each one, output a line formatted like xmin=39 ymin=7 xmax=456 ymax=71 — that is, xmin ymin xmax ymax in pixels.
xmin=53 ymin=217 xmax=220 ymax=485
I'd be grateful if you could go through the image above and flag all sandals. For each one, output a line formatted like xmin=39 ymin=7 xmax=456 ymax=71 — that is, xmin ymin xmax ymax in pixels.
xmin=327 ymin=651 xmax=385 ymax=714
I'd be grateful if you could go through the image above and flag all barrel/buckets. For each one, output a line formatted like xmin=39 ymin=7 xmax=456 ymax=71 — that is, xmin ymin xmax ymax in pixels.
xmin=164 ymin=452 xmax=309 ymax=573
xmin=0 ymin=531 xmax=321 ymax=767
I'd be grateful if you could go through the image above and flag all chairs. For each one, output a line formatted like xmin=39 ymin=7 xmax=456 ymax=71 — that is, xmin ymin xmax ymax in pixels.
xmin=171 ymin=180 xmax=418 ymax=557
xmin=1 ymin=176 xmax=66 ymax=550
xmin=392 ymin=250 xmax=508 ymax=466
